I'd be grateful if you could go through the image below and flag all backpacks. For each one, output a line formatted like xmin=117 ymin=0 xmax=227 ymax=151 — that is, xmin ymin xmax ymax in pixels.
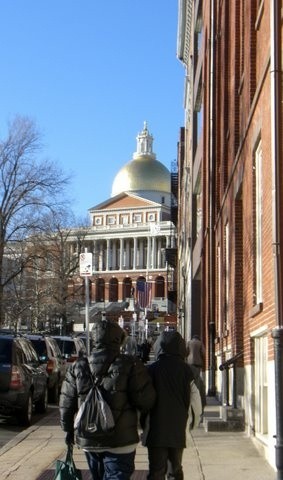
xmin=73 ymin=352 xmax=120 ymax=437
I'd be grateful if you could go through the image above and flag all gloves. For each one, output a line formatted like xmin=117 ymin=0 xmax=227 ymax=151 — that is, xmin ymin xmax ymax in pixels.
xmin=66 ymin=431 xmax=75 ymax=445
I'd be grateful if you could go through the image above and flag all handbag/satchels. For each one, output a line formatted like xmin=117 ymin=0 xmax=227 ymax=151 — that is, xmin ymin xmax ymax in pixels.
xmin=53 ymin=442 xmax=81 ymax=480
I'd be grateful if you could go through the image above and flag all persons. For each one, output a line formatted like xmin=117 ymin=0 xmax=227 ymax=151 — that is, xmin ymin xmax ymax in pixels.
xmin=121 ymin=335 xmax=207 ymax=378
xmin=59 ymin=320 xmax=156 ymax=480
xmin=140 ymin=329 xmax=206 ymax=480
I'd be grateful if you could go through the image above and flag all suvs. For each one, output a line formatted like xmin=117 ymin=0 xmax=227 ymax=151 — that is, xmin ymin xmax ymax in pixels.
xmin=0 ymin=329 xmax=50 ymax=425
xmin=49 ymin=335 xmax=86 ymax=372
xmin=25 ymin=332 xmax=69 ymax=404
